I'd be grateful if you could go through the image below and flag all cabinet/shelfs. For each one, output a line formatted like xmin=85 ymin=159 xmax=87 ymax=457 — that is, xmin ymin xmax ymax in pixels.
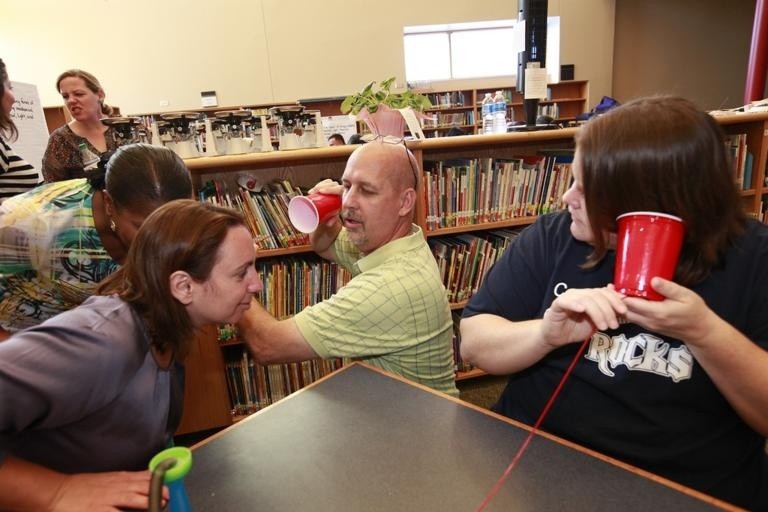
xmin=127 ymin=78 xmax=590 ymax=162
xmin=179 ymin=111 xmax=768 ymax=425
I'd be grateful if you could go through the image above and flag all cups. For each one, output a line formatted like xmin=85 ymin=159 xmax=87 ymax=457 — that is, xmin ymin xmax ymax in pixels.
xmin=613 ymin=213 xmax=685 ymax=302
xmin=284 ymin=192 xmax=345 ymax=232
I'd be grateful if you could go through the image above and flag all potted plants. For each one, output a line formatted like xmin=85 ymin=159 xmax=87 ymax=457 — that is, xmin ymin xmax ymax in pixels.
xmin=338 ymin=76 xmax=436 ymax=142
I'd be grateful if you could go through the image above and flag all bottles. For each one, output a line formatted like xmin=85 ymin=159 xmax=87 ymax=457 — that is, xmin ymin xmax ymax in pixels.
xmin=483 ymin=92 xmax=494 ymax=133
xmin=79 ymin=144 xmax=99 ymax=172
xmin=493 ymin=92 xmax=507 ymax=133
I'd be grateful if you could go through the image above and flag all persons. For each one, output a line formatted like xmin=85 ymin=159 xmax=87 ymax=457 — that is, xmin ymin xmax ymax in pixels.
xmin=347 ymin=134 xmax=368 ymax=145
xmin=460 ymin=94 xmax=768 ymax=512
xmin=42 ymin=70 xmax=121 ymax=183
xmin=0 ymin=143 xmax=192 ymax=343
xmin=0 ymin=58 xmax=39 ymax=206
xmin=0 ymin=199 xmax=263 ymax=512
xmin=237 ymin=138 xmax=460 ymax=402
xmin=328 ymin=134 xmax=346 ymax=146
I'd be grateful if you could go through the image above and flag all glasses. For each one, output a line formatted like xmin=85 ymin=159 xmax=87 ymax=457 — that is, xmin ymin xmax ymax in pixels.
xmin=359 ymin=133 xmax=417 ymax=189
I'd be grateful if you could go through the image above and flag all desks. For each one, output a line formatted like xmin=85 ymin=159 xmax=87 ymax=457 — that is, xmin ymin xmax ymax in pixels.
xmin=136 ymin=360 xmax=750 ymax=512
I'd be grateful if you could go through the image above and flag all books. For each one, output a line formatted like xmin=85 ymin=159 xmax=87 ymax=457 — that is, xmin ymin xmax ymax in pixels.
xmin=452 ymin=308 xmax=478 ymax=375
xmin=423 ymin=90 xmax=559 ymax=128
xmin=427 ymin=226 xmax=533 ymax=304
xmin=221 ymin=344 xmax=350 ymax=417
xmin=216 ymin=249 xmax=360 ymax=343
xmin=421 ymin=153 xmax=575 ymax=232
xmin=194 ymin=178 xmax=313 ymax=250
xmin=723 ymin=133 xmax=754 ymax=191
